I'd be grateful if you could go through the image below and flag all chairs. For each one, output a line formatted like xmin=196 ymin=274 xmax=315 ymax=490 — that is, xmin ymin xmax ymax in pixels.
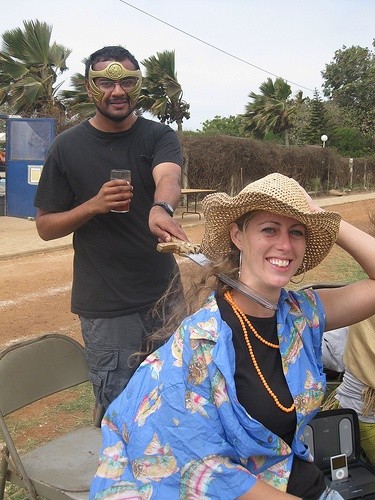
xmin=0 ymin=334 xmax=102 ymax=500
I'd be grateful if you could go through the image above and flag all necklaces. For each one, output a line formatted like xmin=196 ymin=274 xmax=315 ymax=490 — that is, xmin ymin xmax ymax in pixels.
xmin=224 ymin=291 xmax=296 ymax=412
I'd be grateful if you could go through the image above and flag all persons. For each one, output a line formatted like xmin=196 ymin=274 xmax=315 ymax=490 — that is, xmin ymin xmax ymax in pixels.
xmin=88 ymin=172 xmax=375 ymax=500
xmin=33 ymin=46 xmax=189 ymax=428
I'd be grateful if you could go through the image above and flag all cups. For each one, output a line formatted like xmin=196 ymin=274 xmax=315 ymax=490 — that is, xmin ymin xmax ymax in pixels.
xmin=110 ymin=169 xmax=131 ymax=212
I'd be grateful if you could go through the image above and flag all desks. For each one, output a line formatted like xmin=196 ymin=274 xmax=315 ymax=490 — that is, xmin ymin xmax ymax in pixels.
xmin=181 ymin=188 xmax=217 ymax=219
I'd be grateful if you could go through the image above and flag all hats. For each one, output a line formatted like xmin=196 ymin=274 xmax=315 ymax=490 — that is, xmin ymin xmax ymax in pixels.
xmin=200 ymin=172 xmax=340 ymax=276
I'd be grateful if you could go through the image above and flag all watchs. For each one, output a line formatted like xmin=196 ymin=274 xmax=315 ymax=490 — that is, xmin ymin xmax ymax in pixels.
xmin=152 ymin=202 xmax=174 ymax=217
